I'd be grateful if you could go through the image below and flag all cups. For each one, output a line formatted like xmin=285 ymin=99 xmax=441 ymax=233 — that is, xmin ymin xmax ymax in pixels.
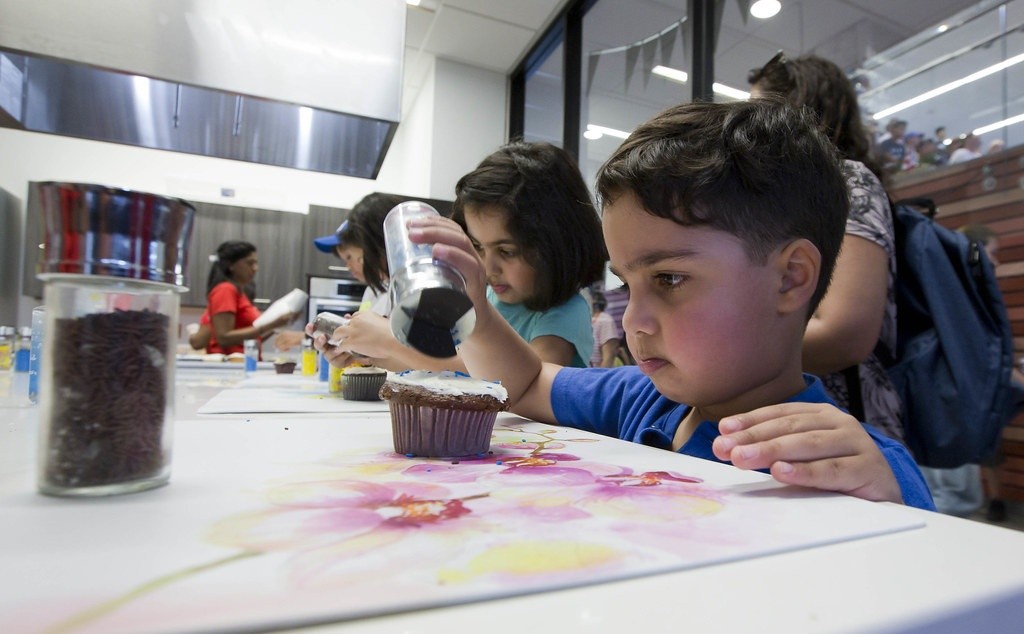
xmin=384 ymin=201 xmax=477 ymax=355
xmin=29 ymin=305 xmax=45 ymax=407
xmin=311 ymin=311 xmax=369 ymax=357
xmin=36 ymin=272 xmax=189 ymax=497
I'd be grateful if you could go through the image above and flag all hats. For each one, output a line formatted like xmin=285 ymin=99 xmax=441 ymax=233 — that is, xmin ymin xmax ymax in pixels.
xmin=886 ymin=118 xmax=907 ymax=130
xmin=314 ymin=218 xmax=350 ymax=253
xmin=904 ymin=131 xmax=923 ymax=140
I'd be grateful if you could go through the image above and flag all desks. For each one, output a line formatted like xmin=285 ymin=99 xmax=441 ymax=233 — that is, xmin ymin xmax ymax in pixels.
xmin=1 ymin=345 xmax=1024 ymax=634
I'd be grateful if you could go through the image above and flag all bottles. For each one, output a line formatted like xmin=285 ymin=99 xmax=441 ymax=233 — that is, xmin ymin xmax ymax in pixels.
xmin=301 ymin=338 xmax=361 ymax=393
xmin=0 ymin=326 xmax=31 ymax=373
xmin=244 ymin=339 xmax=259 ymax=371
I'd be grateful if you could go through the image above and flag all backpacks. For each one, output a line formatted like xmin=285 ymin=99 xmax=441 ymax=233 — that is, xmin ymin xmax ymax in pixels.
xmin=820 ymin=156 xmax=1013 ymax=470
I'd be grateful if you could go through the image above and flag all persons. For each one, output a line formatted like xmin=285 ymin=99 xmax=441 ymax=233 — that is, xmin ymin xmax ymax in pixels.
xmin=746 ymin=49 xmax=985 ymax=518
xmin=871 ymin=117 xmax=1005 ymax=177
xmin=188 ymin=141 xmax=638 ymax=440
xmin=407 ymin=94 xmax=938 ymax=517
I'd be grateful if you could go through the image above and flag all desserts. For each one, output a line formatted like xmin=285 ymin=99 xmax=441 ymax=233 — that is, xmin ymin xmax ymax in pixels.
xmin=379 ymin=368 xmax=509 ymax=457
xmin=273 ymin=358 xmax=297 ymax=374
xmin=225 ymin=352 xmax=245 ymax=362
xmin=340 ymin=364 xmax=388 ymax=402
